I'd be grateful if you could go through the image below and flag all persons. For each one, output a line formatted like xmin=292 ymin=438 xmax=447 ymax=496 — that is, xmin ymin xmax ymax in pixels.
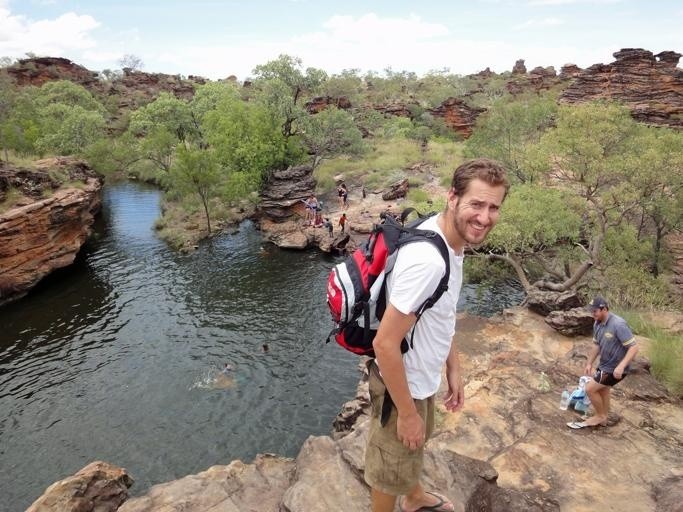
xmin=304 ymin=179 xmax=366 ymax=237
xmin=364 ymin=157 xmax=511 ymax=512
xmin=582 ymin=297 xmax=638 ymax=427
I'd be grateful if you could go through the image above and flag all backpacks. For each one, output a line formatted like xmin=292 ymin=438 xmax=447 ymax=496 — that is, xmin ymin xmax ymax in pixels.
xmin=326 ymin=208 xmax=448 ymax=358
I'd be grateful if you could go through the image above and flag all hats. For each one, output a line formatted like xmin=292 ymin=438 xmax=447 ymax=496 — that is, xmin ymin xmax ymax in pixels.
xmin=588 ymin=298 xmax=607 ymax=308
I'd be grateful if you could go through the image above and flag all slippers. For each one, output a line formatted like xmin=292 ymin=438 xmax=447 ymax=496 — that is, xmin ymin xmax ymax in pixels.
xmin=567 ymin=420 xmax=585 ymax=428
xmin=402 ymin=491 xmax=454 ymax=512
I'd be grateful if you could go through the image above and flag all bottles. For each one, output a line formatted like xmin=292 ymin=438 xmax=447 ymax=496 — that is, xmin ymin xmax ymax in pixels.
xmin=558 ymin=388 xmax=569 ymax=411
